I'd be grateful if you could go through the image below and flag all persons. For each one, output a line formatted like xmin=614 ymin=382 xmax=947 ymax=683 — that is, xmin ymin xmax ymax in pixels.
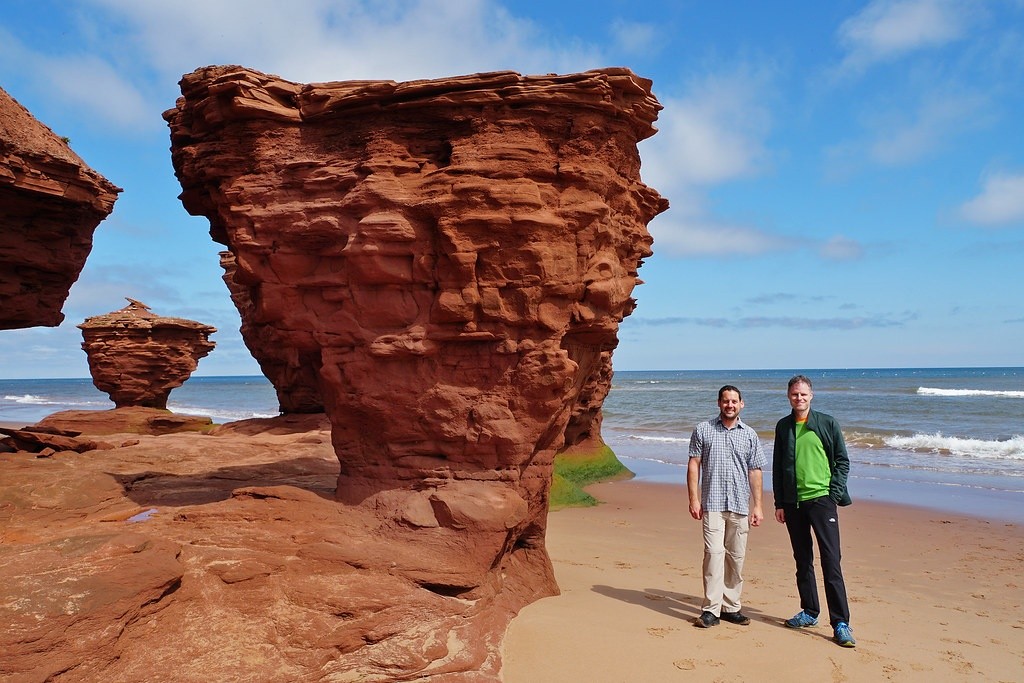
xmin=686 ymin=385 xmax=767 ymax=628
xmin=772 ymin=374 xmax=856 ymax=648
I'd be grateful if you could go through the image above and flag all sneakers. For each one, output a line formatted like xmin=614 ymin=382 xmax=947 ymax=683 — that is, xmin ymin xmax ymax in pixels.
xmin=785 ymin=611 xmax=818 ymax=628
xmin=833 ymin=622 xmax=856 ymax=646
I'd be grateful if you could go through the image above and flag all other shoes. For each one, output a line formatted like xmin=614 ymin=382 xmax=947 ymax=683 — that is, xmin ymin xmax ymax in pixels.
xmin=695 ymin=611 xmax=720 ymax=627
xmin=720 ymin=610 xmax=751 ymax=624
